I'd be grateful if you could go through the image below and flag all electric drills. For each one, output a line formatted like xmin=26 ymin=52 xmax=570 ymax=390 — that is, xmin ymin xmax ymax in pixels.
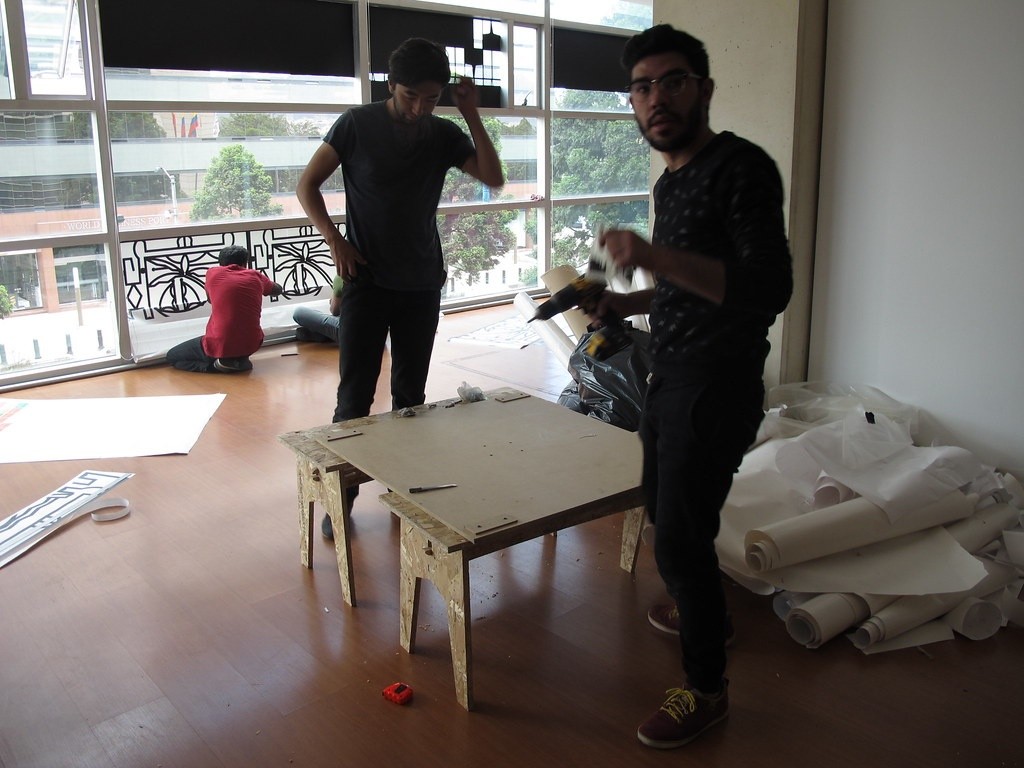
xmin=524 ymin=274 xmax=635 ymax=360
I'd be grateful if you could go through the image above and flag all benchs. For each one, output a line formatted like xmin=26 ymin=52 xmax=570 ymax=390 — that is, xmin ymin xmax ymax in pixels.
xmin=378 ymin=492 xmax=648 ymax=711
xmin=277 ymin=387 xmax=527 ymax=608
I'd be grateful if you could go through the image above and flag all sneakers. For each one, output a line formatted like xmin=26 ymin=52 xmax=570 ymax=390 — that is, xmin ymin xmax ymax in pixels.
xmin=648 ymin=603 xmax=736 ymax=646
xmin=637 ymin=677 xmax=730 ymax=748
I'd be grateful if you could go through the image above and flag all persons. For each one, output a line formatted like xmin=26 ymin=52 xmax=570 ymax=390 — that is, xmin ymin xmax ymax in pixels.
xmin=295 ymin=38 xmax=504 ymax=540
xmin=293 ymin=275 xmax=343 ymax=342
xmin=167 ymin=246 xmax=282 ymax=374
xmin=581 ymin=24 xmax=792 ymax=749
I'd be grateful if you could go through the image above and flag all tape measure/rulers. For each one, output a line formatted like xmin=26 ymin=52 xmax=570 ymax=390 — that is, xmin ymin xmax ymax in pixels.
xmin=382 ymin=682 xmax=415 ymax=704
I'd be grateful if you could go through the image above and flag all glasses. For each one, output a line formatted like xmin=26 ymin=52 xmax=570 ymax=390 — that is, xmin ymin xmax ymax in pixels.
xmin=624 ymin=68 xmax=703 ymax=103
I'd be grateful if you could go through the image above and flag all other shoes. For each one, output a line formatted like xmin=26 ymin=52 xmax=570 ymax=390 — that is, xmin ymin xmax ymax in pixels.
xmin=213 ymin=357 xmax=253 ymax=371
xmin=321 ymin=503 xmax=353 ymax=542
